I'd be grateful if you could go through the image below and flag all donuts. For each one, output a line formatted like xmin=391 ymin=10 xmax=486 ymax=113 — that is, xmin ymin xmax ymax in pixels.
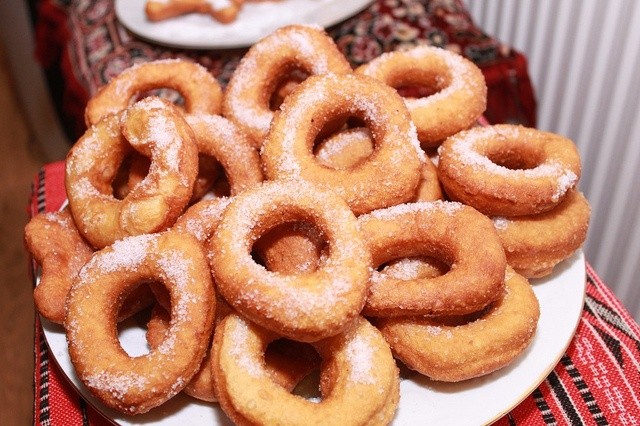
xmin=25 ymin=24 xmax=593 ymax=426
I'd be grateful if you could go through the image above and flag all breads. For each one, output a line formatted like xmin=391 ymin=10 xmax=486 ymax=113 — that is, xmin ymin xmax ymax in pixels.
xmin=143 ymin=0 xmax=288 ymax=23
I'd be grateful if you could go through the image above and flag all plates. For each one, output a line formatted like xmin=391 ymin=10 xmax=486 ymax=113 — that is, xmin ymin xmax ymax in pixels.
xmin=34 ymin=197 xmax=587 ymax=425
xmin=113 ymin=0 xmax=373 ymax=52
